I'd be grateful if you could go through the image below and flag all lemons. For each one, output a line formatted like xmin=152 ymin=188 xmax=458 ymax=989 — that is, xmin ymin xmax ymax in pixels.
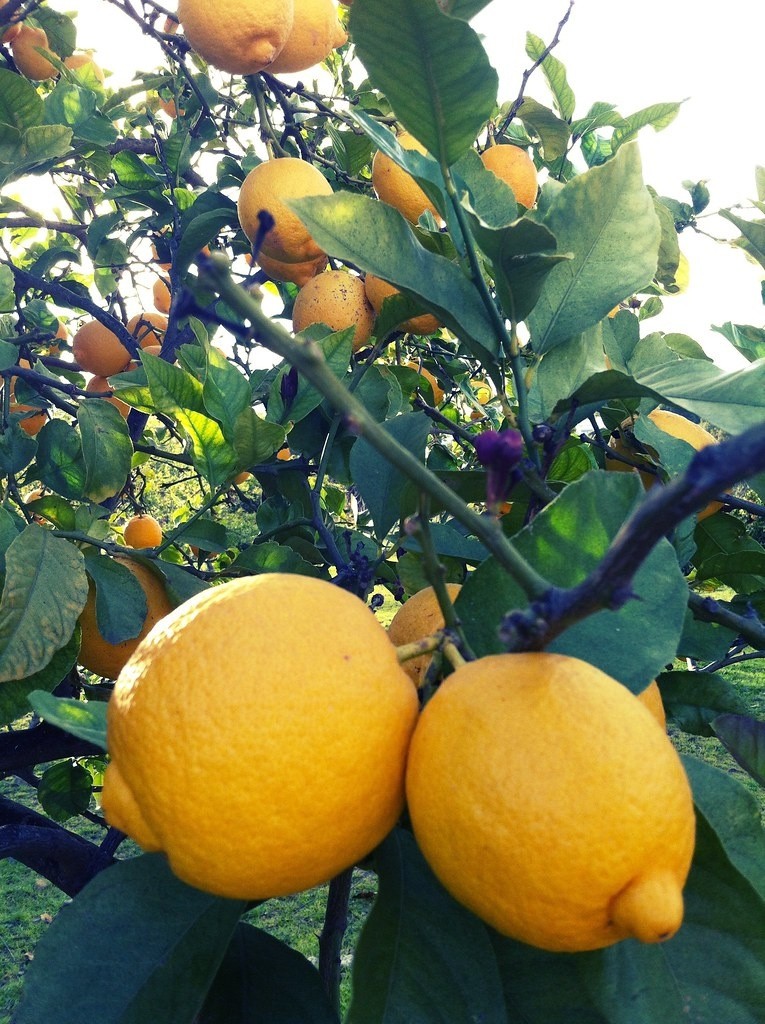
xmin=100 ymin=571 xmax=415 ymax=901
xmin=405 ymin=651 xmax=694 ymax=952
xmin=0 ymin=0 xmax=733 ymax=735
